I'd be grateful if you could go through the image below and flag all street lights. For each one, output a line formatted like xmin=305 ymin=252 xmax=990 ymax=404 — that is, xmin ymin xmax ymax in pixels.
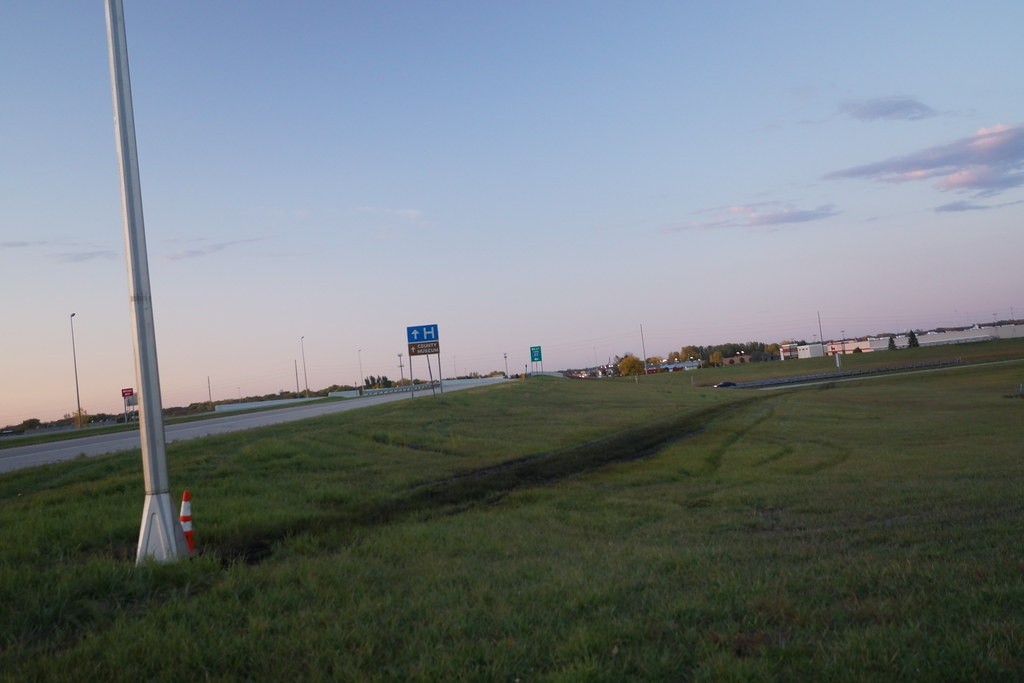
xmin=301 ymin=335 xmax=309 ymax=399
xmin=358 ymin=349 xmax=364 ymax=396
xmin=70 ymin=312 xmax=83 ymax=429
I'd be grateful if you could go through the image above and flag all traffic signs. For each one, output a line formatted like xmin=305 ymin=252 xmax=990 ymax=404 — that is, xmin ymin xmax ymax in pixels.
xmin=406 ymin=324 xmax=440 ymax=356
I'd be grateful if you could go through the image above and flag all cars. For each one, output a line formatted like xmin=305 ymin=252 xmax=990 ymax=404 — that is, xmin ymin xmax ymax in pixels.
xmin=714 ymin=380 xmax=736 ymax=388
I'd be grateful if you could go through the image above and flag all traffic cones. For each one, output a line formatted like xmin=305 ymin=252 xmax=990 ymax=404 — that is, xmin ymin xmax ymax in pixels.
xmin=178 ymin=488 xmax=194 ymax=557
